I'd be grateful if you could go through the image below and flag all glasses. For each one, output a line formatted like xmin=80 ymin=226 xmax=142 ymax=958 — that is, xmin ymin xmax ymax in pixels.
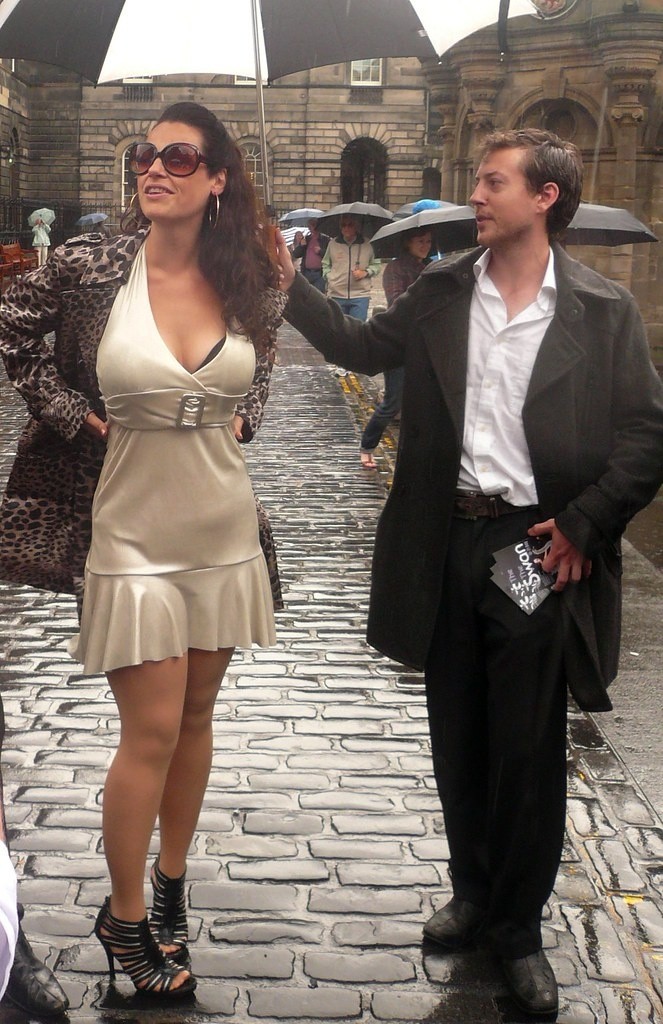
xmin=128 ymin=142 xmax=207 ymax=177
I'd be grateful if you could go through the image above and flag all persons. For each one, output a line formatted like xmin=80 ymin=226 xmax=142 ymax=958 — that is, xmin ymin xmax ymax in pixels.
xmin=31 ymin=217 xmax=51 ymax=268
xmin=359 ymin=225 xmax=437 ymax=469
xmin=262 ymin=125 xmax=663 ymax=1012
xmin=0 ymin=801 xmax=70 ymax=1022
xmin=286 ymin=214 xmax=383 ymax=379
xmin=92 ymin=221 xmax=113 ymax=238
xmin=0 ymin=100 xmax=290 ymax=1003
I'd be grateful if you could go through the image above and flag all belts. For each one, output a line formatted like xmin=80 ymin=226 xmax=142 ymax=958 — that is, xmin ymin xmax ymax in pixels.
xmin=455 ymin=491 xmax=539 ymax=516
xmin=305 ymin=268 xmax=321 ymax=272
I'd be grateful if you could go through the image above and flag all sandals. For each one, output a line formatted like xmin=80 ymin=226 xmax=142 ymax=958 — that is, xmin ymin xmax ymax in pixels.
xmin=361 ymin=454 xmax=377 ymax=468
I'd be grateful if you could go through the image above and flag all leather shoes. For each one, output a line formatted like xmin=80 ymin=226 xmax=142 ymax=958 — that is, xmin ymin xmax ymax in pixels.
xmin=500 ymin=944 xmax=559 ymax=1017
xmin=15 ymin=902 xmax=27 ymax=921
xmin=422 ymin=894 xmax=480 ymax=946
xmin=0 ymin=921 xmax=70 ymax=1018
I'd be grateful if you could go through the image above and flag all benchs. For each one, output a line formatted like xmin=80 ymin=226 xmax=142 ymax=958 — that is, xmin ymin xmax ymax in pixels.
xmin=0 ymin=240 xmax=40 ymax=290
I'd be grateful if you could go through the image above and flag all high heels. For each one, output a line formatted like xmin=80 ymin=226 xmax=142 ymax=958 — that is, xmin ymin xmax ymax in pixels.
xmin=92 ymin=895 xmax=197 ymax=999
xmin=149 ymin=852 xmax=188 ymax=962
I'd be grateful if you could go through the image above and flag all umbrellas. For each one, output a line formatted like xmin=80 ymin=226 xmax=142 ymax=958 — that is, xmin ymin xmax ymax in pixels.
xmin=390 ymin=198 xmax=462 ymax=222
xmin=366 ymin=204 xmax=478 ymax=260
xmin=28 ymin=207 xmax=56 ymax=226
xmin=0 ymin=0 xmax=548 ymax=227
xmin=276 ymin=207 xmax=324 ymax=236
xmin=554 ymin=201 xmax=659 ymax=248
xmin=75 ymin=213 xmax=109 ymax=227
xmin=312 ymin=200 xmax=396 ymax=269
